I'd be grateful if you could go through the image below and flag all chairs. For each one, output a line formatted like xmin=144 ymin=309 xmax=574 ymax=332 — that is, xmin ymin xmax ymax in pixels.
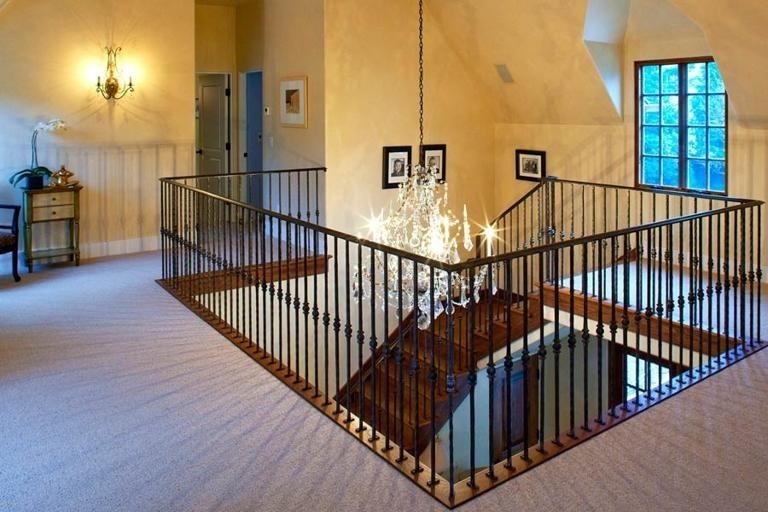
xmin=0 ymin=204 xmax=21 ymax=281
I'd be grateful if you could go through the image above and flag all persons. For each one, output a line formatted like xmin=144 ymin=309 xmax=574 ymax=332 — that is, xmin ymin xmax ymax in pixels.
xmin=426 ymin=156 xmax=440 ymax=175
xmin=391 ymin=159 xmax=404 ymax=177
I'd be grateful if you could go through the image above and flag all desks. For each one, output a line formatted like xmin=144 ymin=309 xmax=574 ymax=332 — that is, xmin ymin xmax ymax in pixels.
xmin=18 ymin=184 xmax=85 ymax=273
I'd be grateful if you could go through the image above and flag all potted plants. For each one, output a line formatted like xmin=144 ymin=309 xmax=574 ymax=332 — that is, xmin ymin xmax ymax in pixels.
xmin=7 ymin=164 xmax=55 ymax=190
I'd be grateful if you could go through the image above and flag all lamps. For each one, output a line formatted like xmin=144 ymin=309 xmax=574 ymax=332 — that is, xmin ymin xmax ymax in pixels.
xmin=97 ymin=40 xmax=134 ymax=102
xmin=350 ymin=7 xmax=496 ymax=333
xmin=28 ymin=117 xmax=67 ymax=168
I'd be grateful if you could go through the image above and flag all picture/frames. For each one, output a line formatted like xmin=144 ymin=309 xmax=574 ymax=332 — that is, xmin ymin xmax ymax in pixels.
xmin=278 ymin=75 xmax=309 ymax=130
xmin=514 ymin=148 xmax=547 ymax=182
xmin=381 ymin=144 xmax=447 ymax=189
